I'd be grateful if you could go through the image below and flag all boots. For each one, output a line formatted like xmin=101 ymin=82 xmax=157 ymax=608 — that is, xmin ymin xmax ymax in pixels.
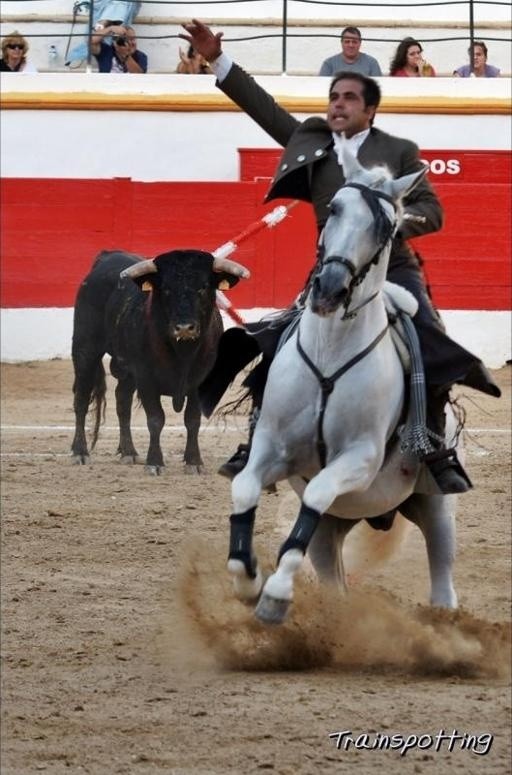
xmin=219 ymin=422 xmax=254 ymax=479
xmin=422 ymin=412 xmax=468 ymax=492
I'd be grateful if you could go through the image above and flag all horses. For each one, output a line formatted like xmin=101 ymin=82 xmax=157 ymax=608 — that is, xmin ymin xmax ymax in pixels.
xmin=219 ymin=130 xmax=468 ymax=632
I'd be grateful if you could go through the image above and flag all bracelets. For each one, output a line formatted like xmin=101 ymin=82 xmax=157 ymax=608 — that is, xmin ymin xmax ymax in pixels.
xmin=122 ymin=54 xmax=130 ymax=64
xmin=202 ymin=62 xmax=210 ymax=69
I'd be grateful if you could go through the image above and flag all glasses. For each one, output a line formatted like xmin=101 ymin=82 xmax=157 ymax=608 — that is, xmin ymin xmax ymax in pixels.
xmin=6 ymin=44 xmax=25 ymax=49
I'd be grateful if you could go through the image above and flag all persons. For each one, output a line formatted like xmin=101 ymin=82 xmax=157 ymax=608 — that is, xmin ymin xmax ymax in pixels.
xmin=319 ymin=26 xmax=383 ymax=76
xmin=0 ymin=29 xmax=29 ymax=71
xmin=84 ymin=19 xmax=147 ymax=75
xmin=177 ymin=16 xmax=475 ymax=492
xmin=174 ymin=43 xmax=214 ymax=75
xmin=451 ymin=40 xmax=501 ymax=77
xmin=389 ymin=36 xmax=436 ymax=77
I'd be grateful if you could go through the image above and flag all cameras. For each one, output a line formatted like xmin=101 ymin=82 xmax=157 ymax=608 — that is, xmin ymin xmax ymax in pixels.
xmin=116 ymin=35 xmax=129 ymax=47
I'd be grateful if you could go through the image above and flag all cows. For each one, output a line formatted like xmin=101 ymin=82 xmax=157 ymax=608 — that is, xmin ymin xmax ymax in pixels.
xmin=68 ymin=247 xmax=253 ymax=479
